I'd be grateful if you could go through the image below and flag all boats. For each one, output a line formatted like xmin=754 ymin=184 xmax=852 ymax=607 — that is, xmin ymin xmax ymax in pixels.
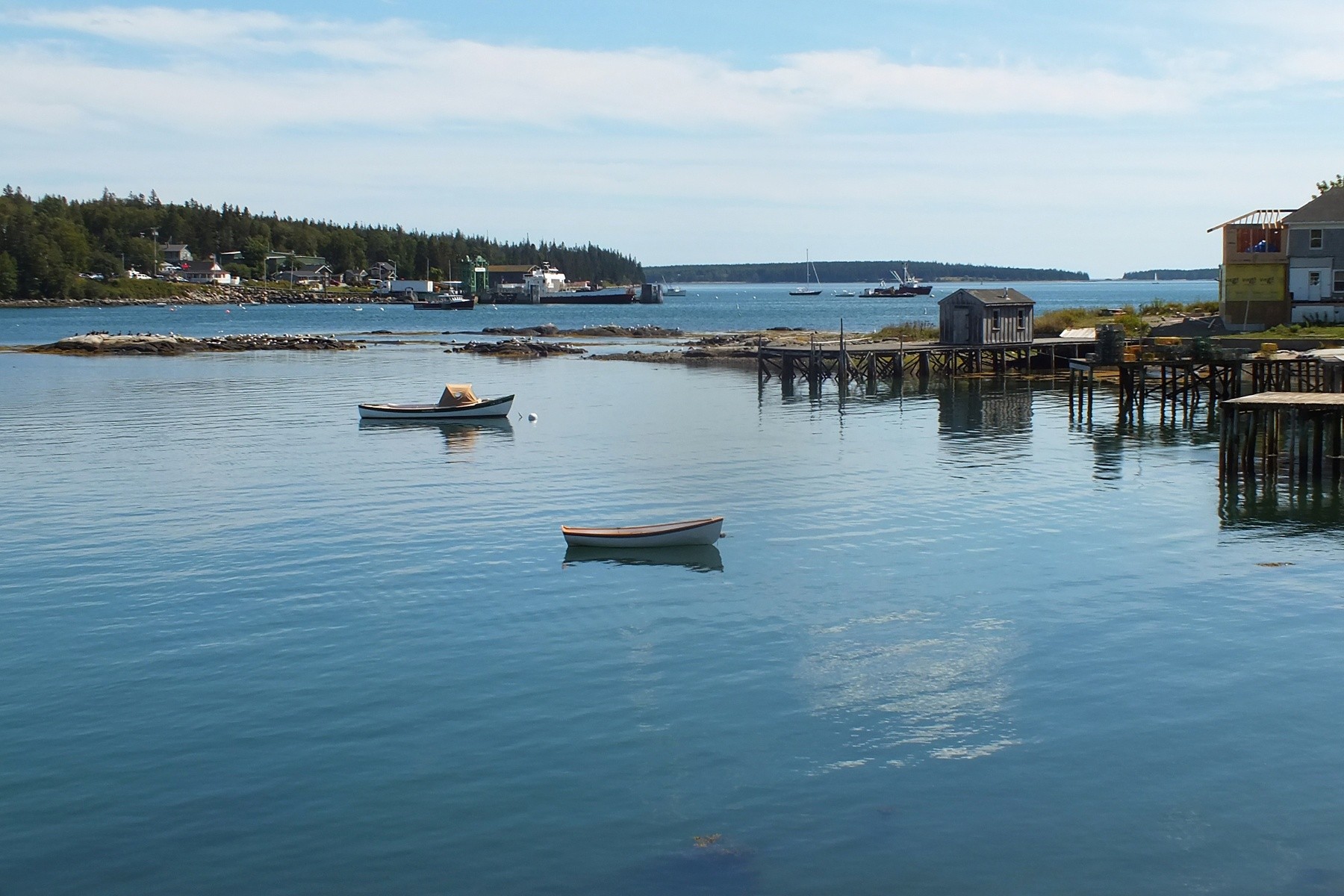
xmin=561 ymin=516 xmax=725 ymax=548
xmin=359 ymin=417 xmax=514 ymax=433
xmin=562 ymin=545 xmax=724 ymax=573
xmin=835 ymin=290 xmax=856 ymax=297
xmin=412 ymin=290 xmax=475 ymax=310
xmin=661 ymin=275 xmax=687 ymax=297
xmin=889 ymin=262 xmax=933 ymax=295
xmin=358 ymin=383 xmax=515 ymax=417
xmin=858 ymin=280 xmax=917 ymax=297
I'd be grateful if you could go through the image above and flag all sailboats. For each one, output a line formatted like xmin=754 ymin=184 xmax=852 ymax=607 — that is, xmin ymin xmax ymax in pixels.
xmin=789 ymin=248 xmax=823 ymax=296
